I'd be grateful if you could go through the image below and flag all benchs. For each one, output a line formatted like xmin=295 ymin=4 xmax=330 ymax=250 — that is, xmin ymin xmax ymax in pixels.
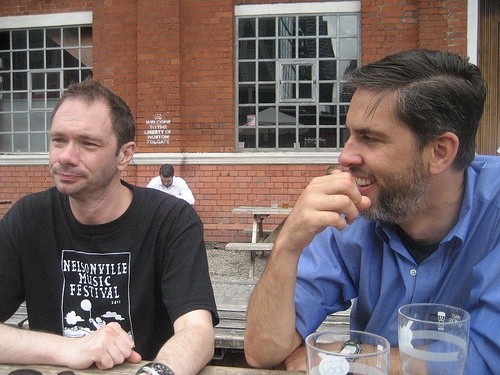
xmin=225 ymin=242 xmax=274 ymax=250
xmin=243 ymin=229 xmax=274 ymax=235
xmin=213 ymin=305 xmax=352 ymax=350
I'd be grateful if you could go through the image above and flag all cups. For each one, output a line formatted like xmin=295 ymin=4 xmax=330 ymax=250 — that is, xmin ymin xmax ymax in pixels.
xmin=397 ymin=303 xmax=471 ymax=375
xmin=270 ymin=195 xmax=277 ymax=209
xmin=305 ymin=329 xmax=391 ymax=375
xmin=281 ymin=196 xmax=289 ymax=209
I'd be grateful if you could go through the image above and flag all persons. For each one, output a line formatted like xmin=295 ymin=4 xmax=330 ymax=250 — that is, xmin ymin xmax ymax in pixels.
xmin=244 ymin=48 xmax=500 ymax=375
xmin=146 ymin=164 xmax=195 ymax=205
xmin=0 ymin=75 xmax=220 ymax=375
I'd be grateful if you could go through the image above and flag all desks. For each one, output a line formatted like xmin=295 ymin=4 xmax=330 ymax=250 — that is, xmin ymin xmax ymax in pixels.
xmin=233 ymin=206 xmax=293 ymax=278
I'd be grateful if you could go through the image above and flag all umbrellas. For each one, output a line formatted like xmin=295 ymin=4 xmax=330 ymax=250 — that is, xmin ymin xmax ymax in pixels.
xmin=238 ymin=107 xmax=309 ymax=144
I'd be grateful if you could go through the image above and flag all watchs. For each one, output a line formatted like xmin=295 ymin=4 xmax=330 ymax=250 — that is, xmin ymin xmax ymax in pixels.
xmin=338 ymin=340 xmax=362 ymax=375
xmin=135 ymin=362 xmax=175 ymax=375
